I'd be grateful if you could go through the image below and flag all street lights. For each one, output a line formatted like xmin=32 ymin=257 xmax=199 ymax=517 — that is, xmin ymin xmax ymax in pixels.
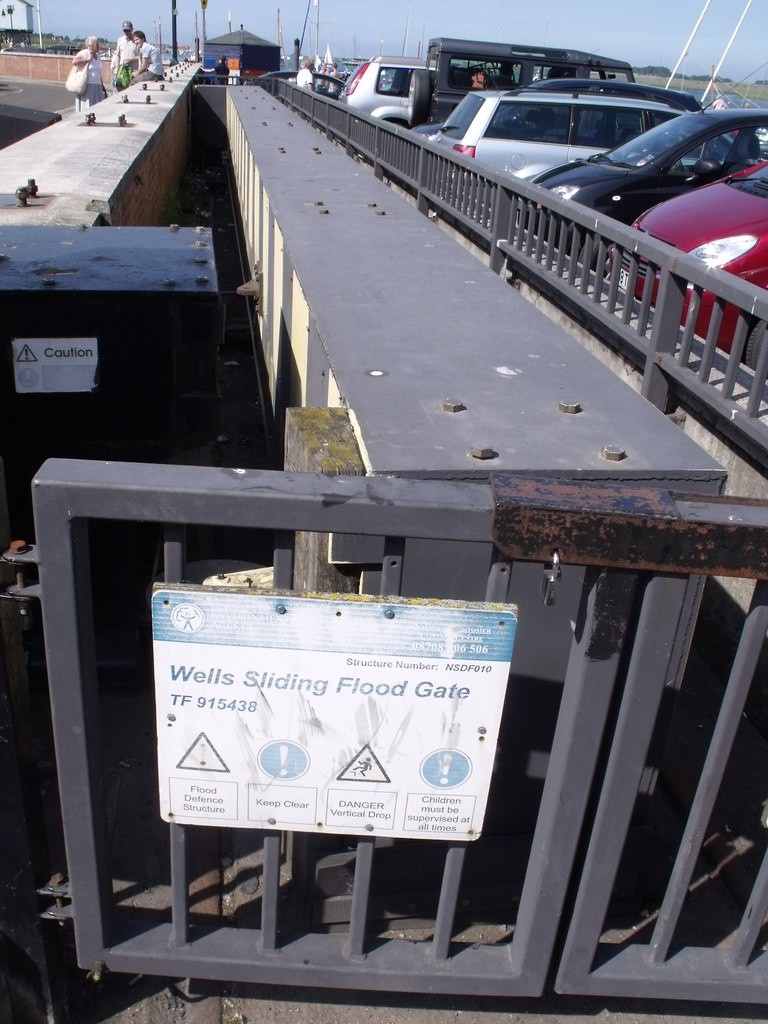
xmin=1 ymin=4 xmax=16 ymax=47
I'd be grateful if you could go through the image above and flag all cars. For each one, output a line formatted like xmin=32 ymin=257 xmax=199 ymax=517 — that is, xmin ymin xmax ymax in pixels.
xmin=323 ymin=60 xmax=362 ymax=81
xmin=243 ymin=69 xmax=346 ymax=101
xmin=0 ymin=44 xmax=84 ymax=56
xmin=524 ymin=104 xmax=768 ymax=278
xmin=612 ymin=158 xmax=768 ymax=380
xmin=674 ymin=84 xmax=768 ymax=154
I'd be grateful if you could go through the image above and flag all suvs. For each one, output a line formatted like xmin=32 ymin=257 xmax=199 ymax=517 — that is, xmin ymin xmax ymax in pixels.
xmin=337 ymin=57 xmax=439 ymax=131
xmin=406 ymin=37 xmax=637 ymax=129
xmin=410 ymin=77 xmax=705 ymax=140
xmin=434 ymin=87 xmax=763 ymax=215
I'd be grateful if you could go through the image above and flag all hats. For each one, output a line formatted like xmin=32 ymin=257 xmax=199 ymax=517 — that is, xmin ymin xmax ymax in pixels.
xmin=122 ymin=21 xmax=132 ymax=30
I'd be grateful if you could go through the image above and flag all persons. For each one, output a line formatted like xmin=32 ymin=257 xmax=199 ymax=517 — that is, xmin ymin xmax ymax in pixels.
xmin=199 ymin=55 xmax=229 ymax=85
xmin=73 ymin=36 xmax=107 ymax=113
xmin=712 ymin=95 xmax=727 ymax=109
xmin=297 ymin=61 xmax=313 ymax=91
xmin=317 ymin=64 xmax=340 ymax=92
xmin=130 ymin=30 xmax=163 ymax=87
xmin=112 ymin=21 xmax=138 ymax=91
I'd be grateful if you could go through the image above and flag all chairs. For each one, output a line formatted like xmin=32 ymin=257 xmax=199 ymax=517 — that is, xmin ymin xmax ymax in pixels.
xmin=548 ymin=69 xmax=563 ymax=80
xmin=726 ymin=134 xmax=759 ymax=177
xmin=489 ymin=67 xmax=514 ymax=87
xmin=589 ymin=118 xmax=601 ymax=137
xmin=510 ymin=109 xmax=541 ymax=140
xmin=613 ymin=122 xmax=637 ymax=147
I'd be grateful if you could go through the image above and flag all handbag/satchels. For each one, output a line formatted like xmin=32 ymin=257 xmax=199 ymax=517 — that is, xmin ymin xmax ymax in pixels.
xmin=65 ymin=49 xmax=91 ymax=94
xmin=116 ymin=62 xmax=133 ymax=89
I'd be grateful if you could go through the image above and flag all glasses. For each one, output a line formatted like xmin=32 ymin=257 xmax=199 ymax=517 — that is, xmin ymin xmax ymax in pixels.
xmin=124 ymin=29 xmax=130 ymax=32
